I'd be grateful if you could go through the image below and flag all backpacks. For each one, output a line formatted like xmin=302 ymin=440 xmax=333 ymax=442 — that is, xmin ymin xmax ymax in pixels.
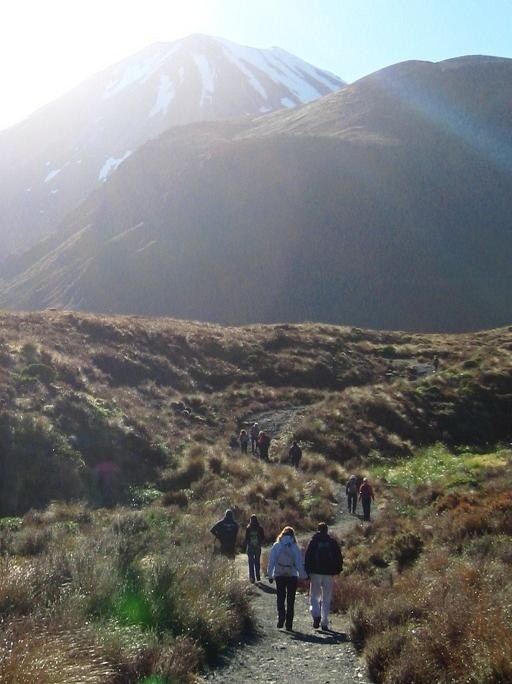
xmin=277 ymin=544 xmax=292 ymax=578
xmin=249 ymin=527 xmax=259 ymax=551
xmin=314 ymin=542 xmax=335 ymax=574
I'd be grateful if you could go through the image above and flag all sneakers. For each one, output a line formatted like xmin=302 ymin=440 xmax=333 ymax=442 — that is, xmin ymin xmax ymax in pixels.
xmin=313 ymin=617 xmax=329 ymax=630
xmin=277 ymin=620 xmax=293 ymax=631
xmin=250 ymin=575 xmax=260 ymax=582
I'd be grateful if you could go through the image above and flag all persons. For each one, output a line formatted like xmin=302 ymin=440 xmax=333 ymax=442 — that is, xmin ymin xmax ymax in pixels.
xmin=238 ymin=428 xmax=248 ymax=455
xmin=287 ymin=439 xmax=303 ymax=468
xmin=266 ymin=524 xmax=309 ymax=628
xmin=257 ymin=430 xmax=272 ymax=463
xmin=357 ymin=476 xmax=375 ymax=522
xmin=344 ymin=473 xmax=359 ymax=516
xmin=241 ymin=513 xmax=266 ymax=584
xmin=248 ymin=423 xmax=261 ymax=454
xmin=303 ymin=520 xmax=344 ymax=632
xmin=209 ymin=508 xmax=239 ymax=563
